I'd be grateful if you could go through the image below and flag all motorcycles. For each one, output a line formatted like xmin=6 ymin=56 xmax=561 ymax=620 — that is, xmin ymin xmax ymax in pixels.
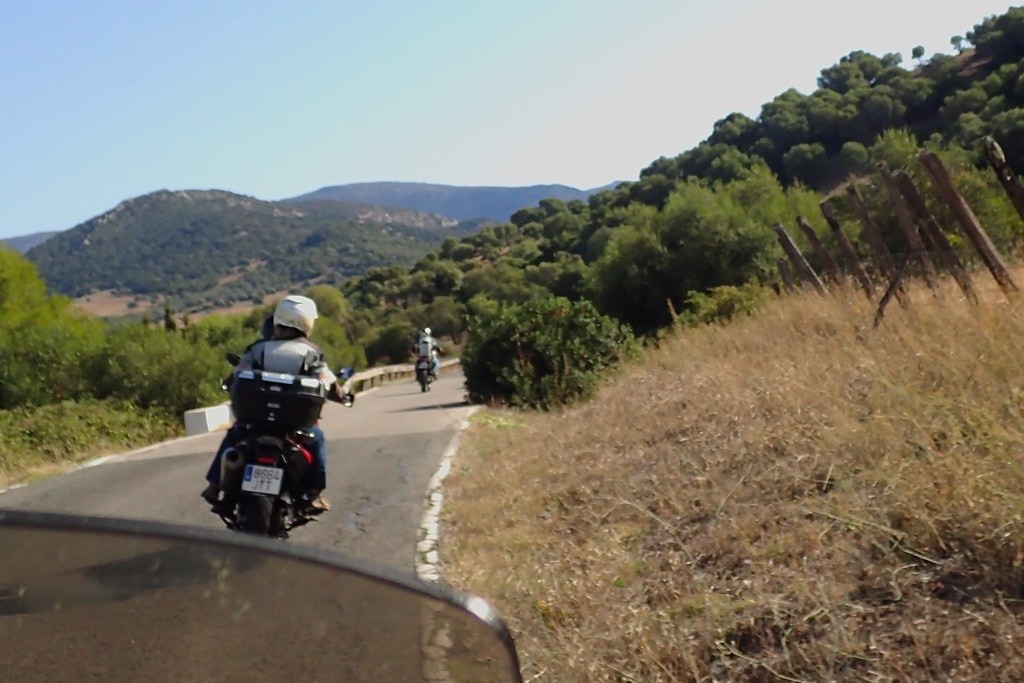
xmin=416 ymin=359 xmax=435 ymax=394
xmin=206 ymin=352 xmax=355 ymax=535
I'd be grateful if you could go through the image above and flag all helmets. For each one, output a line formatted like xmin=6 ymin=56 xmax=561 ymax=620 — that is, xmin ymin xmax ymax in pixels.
xmin=262 ymin=315 xmax=275 ymax=341
xmin=420 ymin=327 xmax=431 ymax=336
xmin=272 ymin=295 xmax=318 ymax=337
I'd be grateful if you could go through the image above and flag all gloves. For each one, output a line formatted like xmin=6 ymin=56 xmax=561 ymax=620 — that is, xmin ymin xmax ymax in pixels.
xmin=344 ymin=388 xmax=354 ymax=404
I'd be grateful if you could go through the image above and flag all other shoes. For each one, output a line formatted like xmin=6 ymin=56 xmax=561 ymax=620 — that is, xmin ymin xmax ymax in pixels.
xmin=301 ymin=496 xmax=330 ymax=514
xmin=200 ymin=487 xmax=219 ymax=505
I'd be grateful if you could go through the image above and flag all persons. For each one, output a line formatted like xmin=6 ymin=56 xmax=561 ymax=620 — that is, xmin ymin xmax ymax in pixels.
xmin=412 ymin=327 xmax=443 ymax=381
xmin=200 ymin=295 xmax=355 ymax=511
